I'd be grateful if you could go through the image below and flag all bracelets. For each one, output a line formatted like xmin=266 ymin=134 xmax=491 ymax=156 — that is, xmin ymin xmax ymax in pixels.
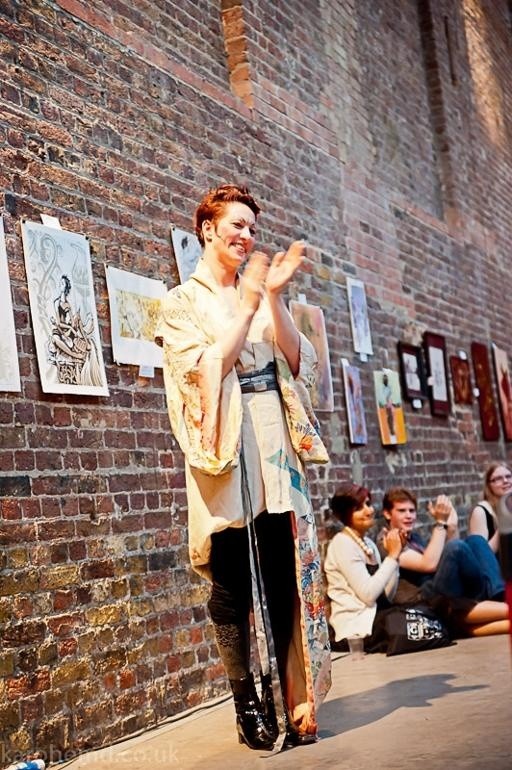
xmin=431 ymin=522 xmax=448 ymax=531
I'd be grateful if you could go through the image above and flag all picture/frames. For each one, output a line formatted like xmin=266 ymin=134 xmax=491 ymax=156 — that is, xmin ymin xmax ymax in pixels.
xmin=396 ymin=340 xmax=427 ymax=403
xmin=450 ymin=356 xmax=473 ymax=406
xmin=423 ymin=331 xmax=451 ymax=416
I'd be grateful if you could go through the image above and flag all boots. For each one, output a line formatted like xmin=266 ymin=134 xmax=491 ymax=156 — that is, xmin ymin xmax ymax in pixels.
xmin=229 ymin=671 xmax=276 ymax=750
xmin=259 ymin=669 xmax=316 ymax=745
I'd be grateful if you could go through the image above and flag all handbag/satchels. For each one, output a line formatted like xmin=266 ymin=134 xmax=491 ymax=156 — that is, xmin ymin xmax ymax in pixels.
xmin=376 ymin=600 xmax=457 ymax=657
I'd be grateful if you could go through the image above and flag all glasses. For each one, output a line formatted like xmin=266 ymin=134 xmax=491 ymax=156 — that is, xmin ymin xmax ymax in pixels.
xmin=489 ymin=475 xmax=511 ymax=483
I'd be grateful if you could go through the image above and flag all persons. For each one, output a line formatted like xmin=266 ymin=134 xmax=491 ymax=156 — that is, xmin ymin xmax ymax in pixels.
xmin=376 ymin=487 xmax=511 ymax=635
xmin=323 ymin=482 xmax=446 ymax=648
xmin=156 ymin=184 xmax=326 ymax=758
xmin=471 ymin=462 xmax=510 ymax=580
xmin=382 ymin=375 xmax=399 ymax=434
